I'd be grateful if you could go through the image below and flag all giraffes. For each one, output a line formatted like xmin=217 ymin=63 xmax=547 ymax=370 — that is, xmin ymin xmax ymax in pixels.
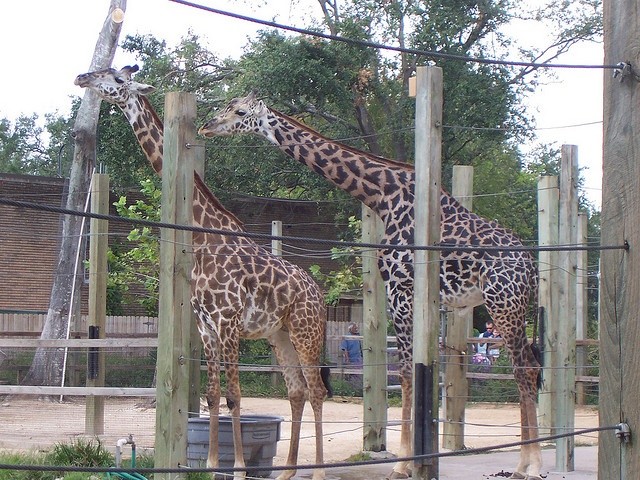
xmin=72 ymin=63 xmax=336 ymax=479
xmin=196 ymin=88 xmax=547 ymax=479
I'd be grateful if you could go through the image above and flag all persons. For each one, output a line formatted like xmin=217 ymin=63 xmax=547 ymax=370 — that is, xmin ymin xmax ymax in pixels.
xmin=488 ymin=326 xmax=502 ymax=363
xmin=341 ymin=323 xmax=363 ymax=380
xmin=480 ymin=320 xmax=505 ymax=358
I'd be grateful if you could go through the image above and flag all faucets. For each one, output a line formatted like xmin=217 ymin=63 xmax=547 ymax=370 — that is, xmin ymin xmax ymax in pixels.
xmin=117 ymin=432 xmax=135 ymax=447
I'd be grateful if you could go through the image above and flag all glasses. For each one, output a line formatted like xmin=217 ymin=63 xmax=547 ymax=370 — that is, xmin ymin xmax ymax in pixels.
xmin=486 ymin=328 xmax=493 ymax=330
